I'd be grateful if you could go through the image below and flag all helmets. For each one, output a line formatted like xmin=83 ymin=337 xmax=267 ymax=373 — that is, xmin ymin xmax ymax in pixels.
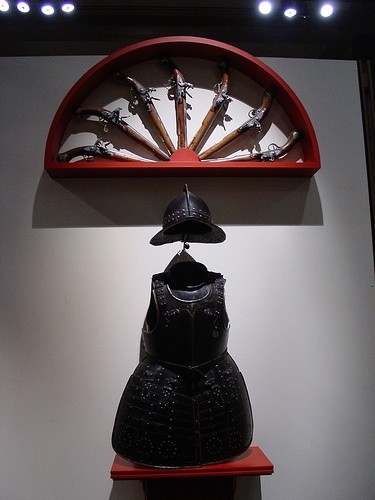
xmin=149 ymin=184 xmax=227 ymax=246
